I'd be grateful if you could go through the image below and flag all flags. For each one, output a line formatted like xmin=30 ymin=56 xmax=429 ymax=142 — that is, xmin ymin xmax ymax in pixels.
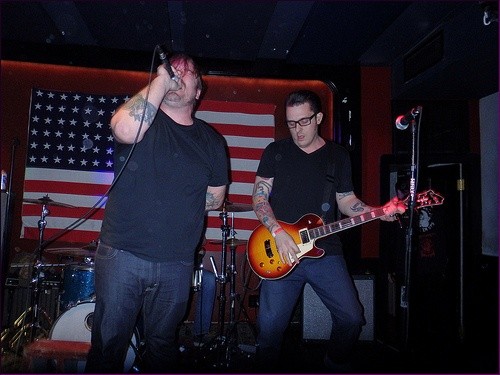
xmin=20 ymin=85 xmax=275 ymax=245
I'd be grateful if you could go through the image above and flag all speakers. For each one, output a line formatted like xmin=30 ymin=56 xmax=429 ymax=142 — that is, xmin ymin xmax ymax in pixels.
xmin=1 ymin=276 xmax=65 ymax=345
xmin=301 ymin=268 xmax=376 ymax=343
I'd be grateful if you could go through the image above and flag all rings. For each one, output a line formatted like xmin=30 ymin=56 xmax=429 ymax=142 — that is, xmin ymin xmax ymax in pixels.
xmin=390 ymin=214 xmax=394 ymax=217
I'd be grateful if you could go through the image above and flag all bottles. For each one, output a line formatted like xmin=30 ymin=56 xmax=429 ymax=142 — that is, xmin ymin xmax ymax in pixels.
xmin=0 ymin=169 xmax=8 ymax=192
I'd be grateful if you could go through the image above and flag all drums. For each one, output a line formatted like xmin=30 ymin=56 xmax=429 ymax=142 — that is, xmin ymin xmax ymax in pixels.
xmin=49 ymin=299 xmax=138 ymax=374
xmin=37 ymin=241 xmax=102 ymax=321
xmin=188 ymin=266 xmax=218 ymax=341
xmin=6 ymin=262 xmax=37 ymax=305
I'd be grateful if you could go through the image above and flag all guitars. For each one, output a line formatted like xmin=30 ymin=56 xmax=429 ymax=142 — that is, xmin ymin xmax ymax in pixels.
xmin=246 ymin=189 xmax=444 ymax=280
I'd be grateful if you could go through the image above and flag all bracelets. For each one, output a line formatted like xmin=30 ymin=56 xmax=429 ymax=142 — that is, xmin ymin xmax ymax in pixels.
xmin=271 ymin=227 xmax=284 ymax=238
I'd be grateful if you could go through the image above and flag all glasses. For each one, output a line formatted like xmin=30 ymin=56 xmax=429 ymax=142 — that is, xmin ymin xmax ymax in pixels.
xmin=284 ymin=113 xmax=317 ymax=129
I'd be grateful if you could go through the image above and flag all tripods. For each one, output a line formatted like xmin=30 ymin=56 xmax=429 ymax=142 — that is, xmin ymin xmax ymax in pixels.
xmin=1 ymin=204 xmax=55 ymax=352
xmin=192 ymin=203 xmax=259 ymax=368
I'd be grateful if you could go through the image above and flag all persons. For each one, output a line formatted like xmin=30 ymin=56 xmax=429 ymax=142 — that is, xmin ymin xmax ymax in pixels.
xmin=85 ymin=55 xmax=230 ymax=374
xmin=252 ymin=91 xmax=406 ymax=375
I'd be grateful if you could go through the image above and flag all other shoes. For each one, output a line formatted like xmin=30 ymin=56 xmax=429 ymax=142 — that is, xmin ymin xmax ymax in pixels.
xmin=192 ymin=334 xmax=211 ymax=348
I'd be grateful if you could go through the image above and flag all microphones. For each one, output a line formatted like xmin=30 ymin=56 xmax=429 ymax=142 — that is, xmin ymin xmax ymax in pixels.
xmin=395 ymin=105 xmax=421 ymax=130
xmin=158 ymin=47 xmax=179 ymax=82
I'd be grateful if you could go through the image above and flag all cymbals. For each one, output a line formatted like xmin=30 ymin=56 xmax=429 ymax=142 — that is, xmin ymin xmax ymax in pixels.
xmin=209 ymin=238 xmax=249 ymax=247
xmin=20 ymin=198 xmax=75 ymax=209
xmin=205 ymin=201 xmax=255 ymax=212
